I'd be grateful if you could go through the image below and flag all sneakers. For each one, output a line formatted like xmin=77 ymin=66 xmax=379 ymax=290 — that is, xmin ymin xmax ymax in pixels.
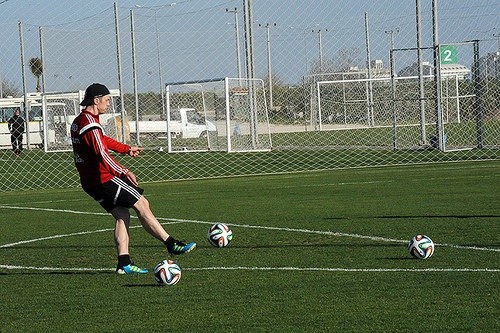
xmin=115 ymin=261 xmax=148 ymax=275
xmin=167 ymin=239 xmax=197 ymax=257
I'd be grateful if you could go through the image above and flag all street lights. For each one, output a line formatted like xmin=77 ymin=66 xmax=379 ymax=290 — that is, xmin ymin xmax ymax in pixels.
xmin=470 ymin=27 xmax=495 ymax=79
xmin=136 ymin=3 xmax=176 ymax=114
xmin=290 ymin=24 xmax=320 ymax=74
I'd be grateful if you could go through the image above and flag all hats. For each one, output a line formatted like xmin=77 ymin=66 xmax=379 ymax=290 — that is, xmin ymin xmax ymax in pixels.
xmin=79 ymin=83 xmax=111 ymax=106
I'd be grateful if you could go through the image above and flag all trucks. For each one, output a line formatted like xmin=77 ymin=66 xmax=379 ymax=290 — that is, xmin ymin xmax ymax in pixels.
xmin=127 ymin=109 xmax=218 ymax=146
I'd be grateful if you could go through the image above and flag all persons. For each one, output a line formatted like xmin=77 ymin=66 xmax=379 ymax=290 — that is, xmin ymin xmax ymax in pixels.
xmin=8 ymin=109 xmax=24 ymax=153
xmin=70 ymin=84 xmax=197 ymax=274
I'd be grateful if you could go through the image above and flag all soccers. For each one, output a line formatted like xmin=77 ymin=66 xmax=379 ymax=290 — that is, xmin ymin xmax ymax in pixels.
xmin=408 ymin=233 xmax=435 ymax=259
xmin=207 ymin=222 xmax=233 ymax=248
xmin=153 ymin=259 xmax=182 ymax=286
